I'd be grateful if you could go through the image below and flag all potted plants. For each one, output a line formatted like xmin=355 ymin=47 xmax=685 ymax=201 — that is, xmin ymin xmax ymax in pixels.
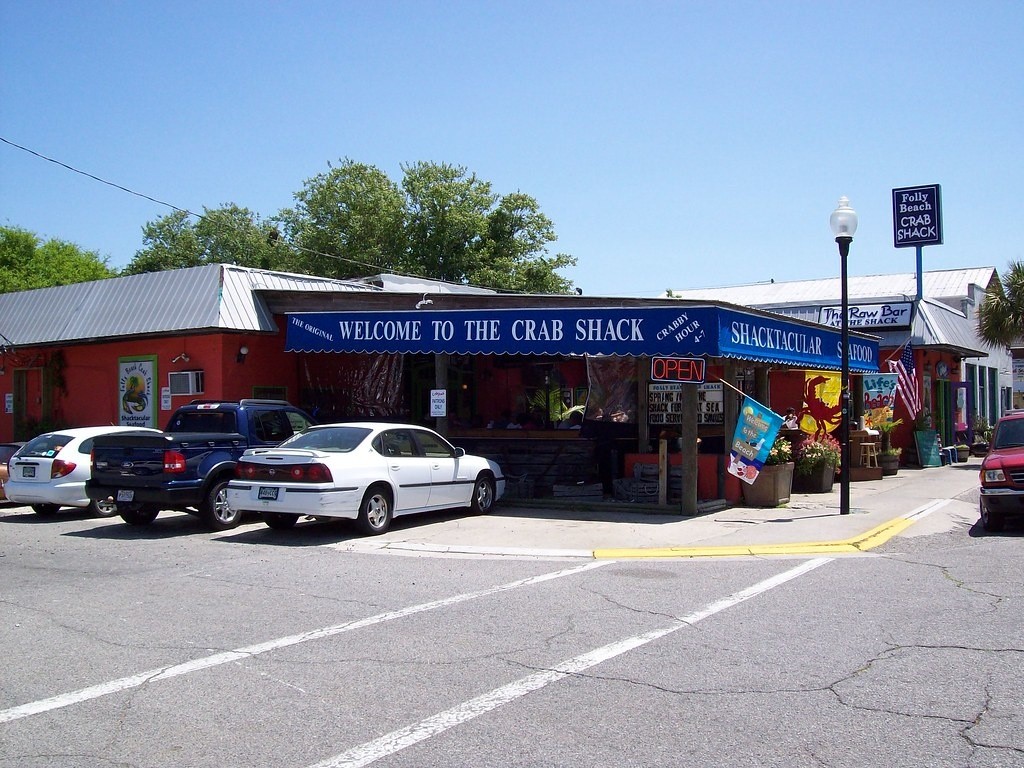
xmin=955 ymin=443 xmax=971 ymax=462
xmin=972 ymin=418 xmax=989 ymax=457
xmin=874 ymin=417 xmax=903 ymax=475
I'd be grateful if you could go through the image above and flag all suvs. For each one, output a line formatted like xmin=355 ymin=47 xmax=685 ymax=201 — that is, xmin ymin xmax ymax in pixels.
xmin=978 ymin=412 xmax=1024 ymax=533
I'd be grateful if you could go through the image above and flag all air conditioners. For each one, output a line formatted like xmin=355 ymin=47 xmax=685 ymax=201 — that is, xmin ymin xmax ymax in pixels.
xmin=168 ymin=370 xmax=204 ymax=395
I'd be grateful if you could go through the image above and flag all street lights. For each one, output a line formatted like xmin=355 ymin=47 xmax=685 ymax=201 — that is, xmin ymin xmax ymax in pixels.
xmin=830 ymin=196 xmax=854 ymax=515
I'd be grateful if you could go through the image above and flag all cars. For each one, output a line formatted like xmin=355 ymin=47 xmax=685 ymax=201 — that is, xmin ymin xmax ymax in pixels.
xmin=226 ymin=422 xmax=506 ymax=536
xmin=0 ymin=442 xmax=32 ymax=500
xmin=4 ymin=425 xmax=174 ymax=519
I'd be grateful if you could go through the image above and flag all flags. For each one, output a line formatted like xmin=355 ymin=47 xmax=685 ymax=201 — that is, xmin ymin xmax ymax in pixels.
xmin=727 ymin=395 xmax=785 ymax=485
xmin=795 ymin=370 xmax=842 ymax=443
xmin=885 ymin=336 xmax=921 ymax=422
xmin=862 ymin=373 xmax=899 ymax=429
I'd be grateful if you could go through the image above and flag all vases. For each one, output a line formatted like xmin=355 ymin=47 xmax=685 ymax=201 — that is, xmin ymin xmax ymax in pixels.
xmin=745 ymin=462 xmax=794 ymax=507
xmin=801 ymin=457 xmax=835 ymax=493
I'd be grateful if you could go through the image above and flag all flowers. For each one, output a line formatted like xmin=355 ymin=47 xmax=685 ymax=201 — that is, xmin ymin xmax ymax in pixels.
xmin=768 ymin=431 xmax=842 ymax=467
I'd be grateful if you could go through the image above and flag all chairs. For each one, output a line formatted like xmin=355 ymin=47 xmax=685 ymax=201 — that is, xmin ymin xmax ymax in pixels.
xmin=385 ymin=443 xmax=401 ymax=456
xmin=268 ymin=426 xmax=285 ymax=441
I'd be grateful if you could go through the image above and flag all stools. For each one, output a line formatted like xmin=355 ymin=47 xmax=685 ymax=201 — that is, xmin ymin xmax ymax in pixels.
xmin=859 ymin=442 xmax=878 ymax=468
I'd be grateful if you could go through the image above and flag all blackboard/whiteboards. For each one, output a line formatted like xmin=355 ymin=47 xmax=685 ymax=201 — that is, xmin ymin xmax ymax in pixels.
xmin=913 ymin=429 xmax=943 ymax=468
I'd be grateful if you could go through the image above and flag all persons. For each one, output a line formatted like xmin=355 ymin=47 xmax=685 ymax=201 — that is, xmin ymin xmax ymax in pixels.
xmin=558 ymin=411 xmax=583 ymax=429
xmin=1014 ymin=404 xmax=1020 ymax=409
xmin=782 ymin=407 xmax=798 ymax=428
xmin=422 ymin=410 xmax=463 ymax=427
xmin=492 ymin=408 xmax=545 ymax=429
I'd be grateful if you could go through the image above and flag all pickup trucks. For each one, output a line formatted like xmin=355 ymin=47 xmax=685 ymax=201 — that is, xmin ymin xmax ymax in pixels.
xmin=85 ymin=400 xmax=320 ymax=531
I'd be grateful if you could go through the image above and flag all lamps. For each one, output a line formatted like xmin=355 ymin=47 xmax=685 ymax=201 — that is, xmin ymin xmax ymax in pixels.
xmin=237 ymin=347 xmax=249 ymax=363
xmin=172 ymin=352 xmax=190 ymax=363
xmin=0 ymin=358 xmax=6 ymax=376
xmin=951 ymin=365 xmax=960 ymax=374
